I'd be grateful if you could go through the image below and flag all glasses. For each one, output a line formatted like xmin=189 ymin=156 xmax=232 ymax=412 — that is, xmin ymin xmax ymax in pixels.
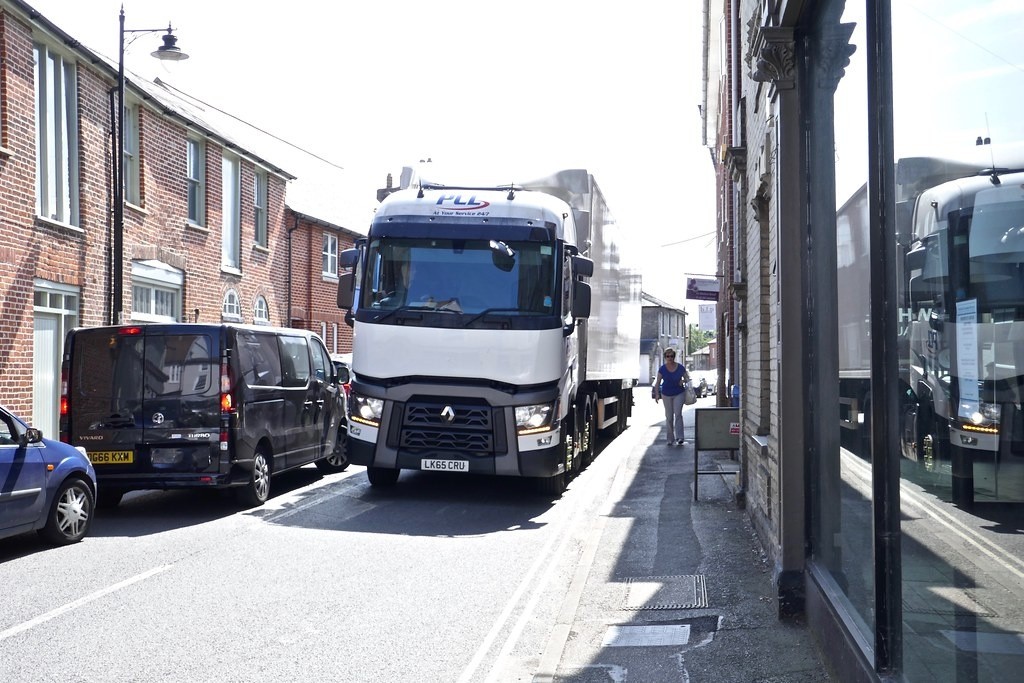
xmin=665 ymin=355 xmax=674 ymax=358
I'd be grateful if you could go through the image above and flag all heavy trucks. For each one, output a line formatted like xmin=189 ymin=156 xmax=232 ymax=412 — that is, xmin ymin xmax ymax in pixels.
xmin=831 ymin=157 xmax=1023 ymax=524
xmin=339 ymin=163 xmax=642 ymax=494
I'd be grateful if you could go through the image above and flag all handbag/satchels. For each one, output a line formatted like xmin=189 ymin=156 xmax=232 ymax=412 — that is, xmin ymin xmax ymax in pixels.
xmin=683 ymin=381 xmax=697 ymax=405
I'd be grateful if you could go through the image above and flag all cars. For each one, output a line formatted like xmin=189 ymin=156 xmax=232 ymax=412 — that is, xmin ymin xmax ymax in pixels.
xmin=697 ymin=370 xmax=717 ymax=395
xmin=688 ymin=373 xmax=708 ymax=397
xmin=652 ymin=375 xmax=692 ymax=398
xmin=329 ymin=352 xmax=354 ymax=402
xmin=0 ymin=405 xmax=98 ymax=551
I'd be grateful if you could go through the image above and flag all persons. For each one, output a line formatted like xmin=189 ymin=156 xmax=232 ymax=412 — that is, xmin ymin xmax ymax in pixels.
xmin=655 ymin=348 xmax=689 ymax=445
xmin=379 ymin=261 xmax=432 ymax=306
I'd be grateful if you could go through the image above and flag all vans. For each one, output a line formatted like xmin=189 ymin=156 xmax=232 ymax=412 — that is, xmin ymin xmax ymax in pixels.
xmin=60 ymin=326 xmax=354 ymax=506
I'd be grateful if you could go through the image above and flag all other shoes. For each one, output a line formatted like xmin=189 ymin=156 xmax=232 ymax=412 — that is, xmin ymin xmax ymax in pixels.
xmin=677 ymin=438 xmax=684 ymax=445
xmin=667 ymin=441 xmax=673 ymax=445
